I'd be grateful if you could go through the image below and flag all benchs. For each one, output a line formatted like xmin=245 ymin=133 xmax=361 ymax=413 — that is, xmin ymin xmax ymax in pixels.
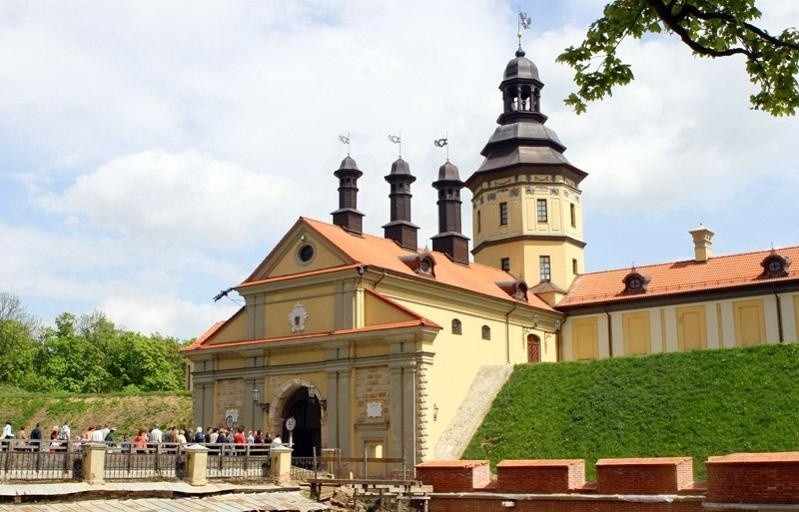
xmin=307 ymin=478 xmax=418 ymax=509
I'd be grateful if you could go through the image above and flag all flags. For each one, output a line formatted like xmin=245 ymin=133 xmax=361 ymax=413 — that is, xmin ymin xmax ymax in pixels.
xmin=388 ymin=135 xmax=400 ymax=144
xmin=434 ymin=138 xmax=447 ymax=147
xmin=519 ymin=5 xmax=531 ymax=29
xmin=339 ymin=135 xmax=350 ymax=145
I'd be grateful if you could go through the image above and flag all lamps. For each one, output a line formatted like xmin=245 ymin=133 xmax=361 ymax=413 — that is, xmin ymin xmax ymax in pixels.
xmin=252 ymin=387 xmax=259 ymax=400
xmin=555 ymin=319 xmax=560 ymax=330
xmin=308 ymin=381 xmax=315 ymax=398
xmin=533 ymin=313 xmax=539 ymax=324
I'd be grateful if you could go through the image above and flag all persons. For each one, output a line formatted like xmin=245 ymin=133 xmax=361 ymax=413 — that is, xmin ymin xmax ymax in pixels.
xmin=0 ymin=420 xmax=281 ymax=455
